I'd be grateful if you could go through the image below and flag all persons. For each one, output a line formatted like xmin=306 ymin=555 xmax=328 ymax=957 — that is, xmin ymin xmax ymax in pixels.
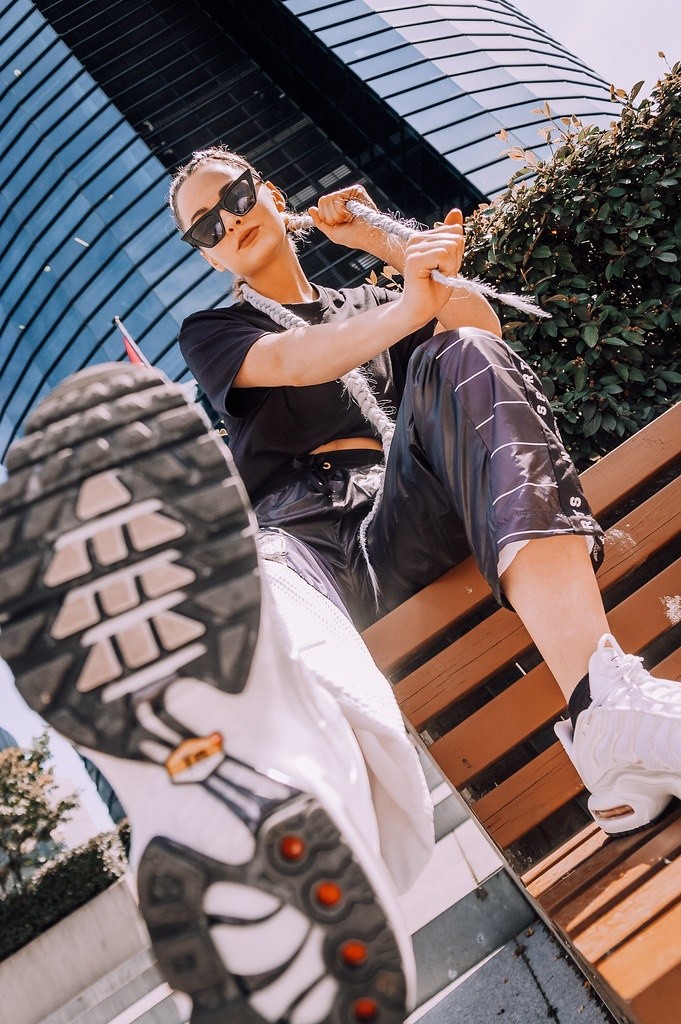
xmin=0 ymin=151 xmax=681 ymax=1024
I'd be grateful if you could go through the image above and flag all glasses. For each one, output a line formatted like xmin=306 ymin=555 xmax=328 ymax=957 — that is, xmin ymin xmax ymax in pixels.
xmin=180 ymin=168 xmax=266 ymax=250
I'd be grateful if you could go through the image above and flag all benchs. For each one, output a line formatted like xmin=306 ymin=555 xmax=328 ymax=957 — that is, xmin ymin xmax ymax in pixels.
xmin=362 ymin=399 xmax=681 ymax=1024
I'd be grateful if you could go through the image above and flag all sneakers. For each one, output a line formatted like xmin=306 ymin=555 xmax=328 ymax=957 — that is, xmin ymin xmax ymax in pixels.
xmin=553 ymin=634 xmax=681 ymax=834
xmin=0 ymin=363 xmax=415 ymax=1024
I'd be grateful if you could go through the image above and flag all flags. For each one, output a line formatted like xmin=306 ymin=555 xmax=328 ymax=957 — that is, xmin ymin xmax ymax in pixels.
xmin=123 ymin=337 xmax=146 ymax=367
